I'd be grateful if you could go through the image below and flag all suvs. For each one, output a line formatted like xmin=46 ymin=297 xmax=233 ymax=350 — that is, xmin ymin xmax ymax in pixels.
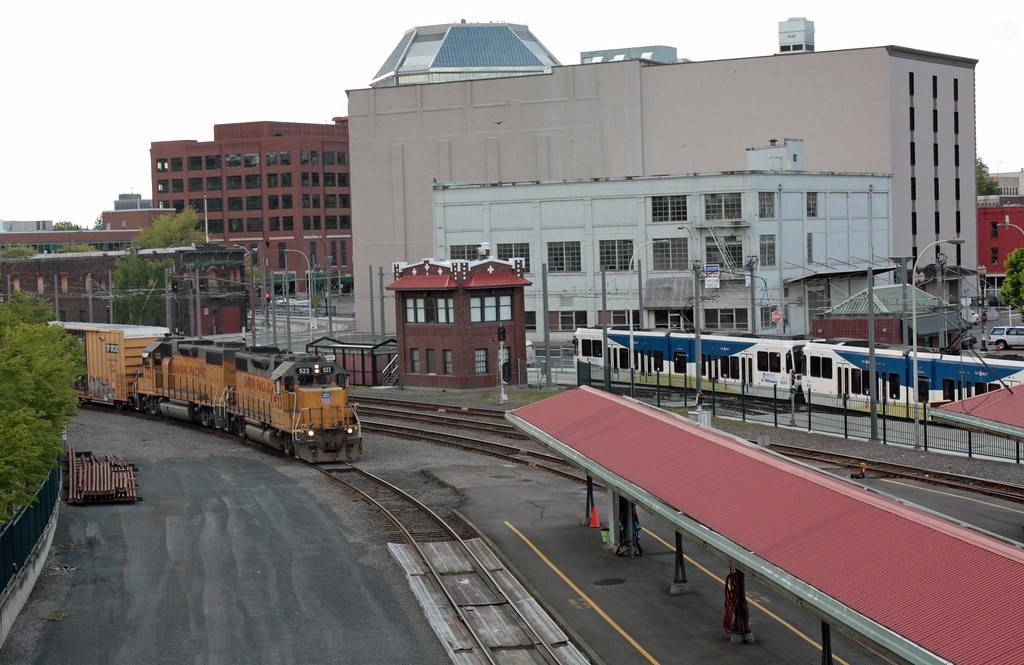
xmin=988 ymin=325 xmax=1024 ymax=349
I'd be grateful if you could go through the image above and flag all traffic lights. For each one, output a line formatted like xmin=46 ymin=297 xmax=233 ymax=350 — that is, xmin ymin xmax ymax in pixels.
xmin=265 ymin=293 xmax=270 ymax=303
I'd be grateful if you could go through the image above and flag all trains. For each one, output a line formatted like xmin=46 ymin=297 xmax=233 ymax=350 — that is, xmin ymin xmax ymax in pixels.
xmin=41 ymin=321 xmax=365 ymax=465
xmin=571 ymin=323 xmax=1019 ymax=425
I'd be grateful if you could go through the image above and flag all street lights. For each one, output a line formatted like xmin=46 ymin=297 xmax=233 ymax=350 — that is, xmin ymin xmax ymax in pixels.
xmin=912 ymin=235 xmax=966 ymax=449
xmin=627 ymin=238 xmax=671 ymax=395
xmin=283 ymin=249 xmax=313 ymax=341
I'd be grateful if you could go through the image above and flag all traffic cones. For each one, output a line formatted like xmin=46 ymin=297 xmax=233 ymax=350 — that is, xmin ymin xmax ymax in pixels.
xmin=588 ymin=504 xmax=602 ymax=528
